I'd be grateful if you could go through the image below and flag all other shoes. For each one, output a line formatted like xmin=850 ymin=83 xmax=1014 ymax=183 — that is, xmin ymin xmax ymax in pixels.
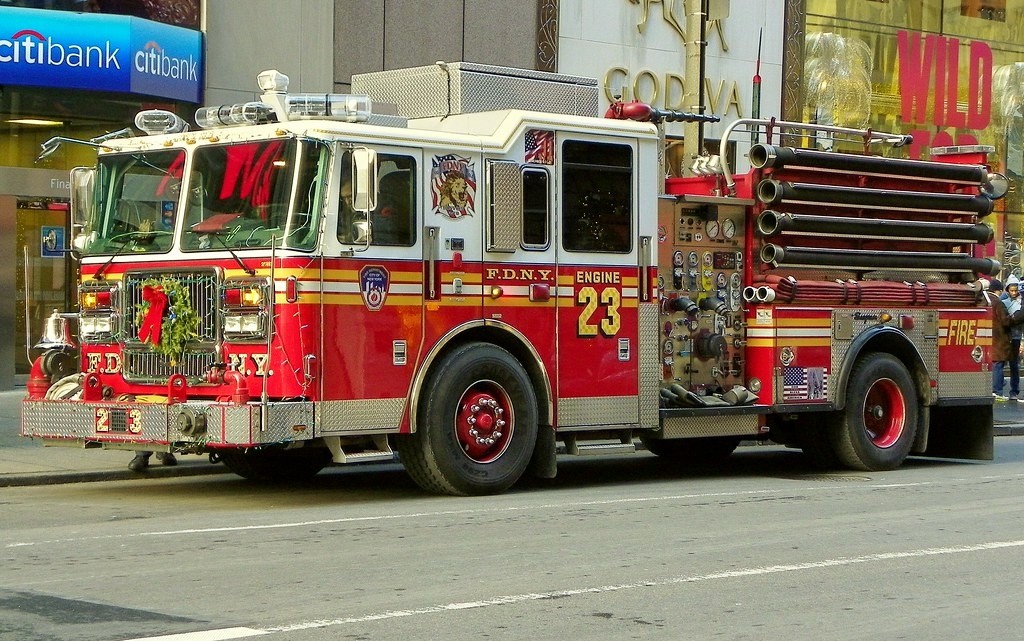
xmin=1017 ymin=398 xmax=1024 ymax=403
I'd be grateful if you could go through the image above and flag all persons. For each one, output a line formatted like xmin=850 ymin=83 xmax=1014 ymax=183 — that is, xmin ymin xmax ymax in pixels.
xmin=988 ymin=274 xmax=1024 ymax=402
xmin=341 ymin=168 xmax=371 ymax=243
xmin=129 ymin=450 xmax=178 ymax=470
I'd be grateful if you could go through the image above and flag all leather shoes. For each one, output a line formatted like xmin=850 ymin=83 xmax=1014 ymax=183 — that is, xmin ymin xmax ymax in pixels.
xmin=1010 ymin=394 xmax=1018 ymax=399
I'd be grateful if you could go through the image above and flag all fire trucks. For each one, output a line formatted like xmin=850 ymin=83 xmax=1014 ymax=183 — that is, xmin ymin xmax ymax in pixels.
xmin=20 ymin=29 xmax=1009 ymax=499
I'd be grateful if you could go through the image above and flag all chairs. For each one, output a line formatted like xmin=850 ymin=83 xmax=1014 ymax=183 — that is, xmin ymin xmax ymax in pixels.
xmin=377 ymin=170 xmax=410 ymax=244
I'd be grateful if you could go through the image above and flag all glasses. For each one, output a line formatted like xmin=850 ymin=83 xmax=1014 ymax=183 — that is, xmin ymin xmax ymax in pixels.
xmin=340 ymin=194 xmax=351 ymax=201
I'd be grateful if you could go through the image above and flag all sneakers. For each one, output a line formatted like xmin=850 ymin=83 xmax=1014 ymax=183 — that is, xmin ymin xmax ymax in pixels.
xmin=992 ymin=392 xmax=1010 ymax=402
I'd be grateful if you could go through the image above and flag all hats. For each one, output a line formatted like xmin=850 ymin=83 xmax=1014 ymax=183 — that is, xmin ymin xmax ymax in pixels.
xmin=1018 ymin=282 xmax=1024 ymax=293
